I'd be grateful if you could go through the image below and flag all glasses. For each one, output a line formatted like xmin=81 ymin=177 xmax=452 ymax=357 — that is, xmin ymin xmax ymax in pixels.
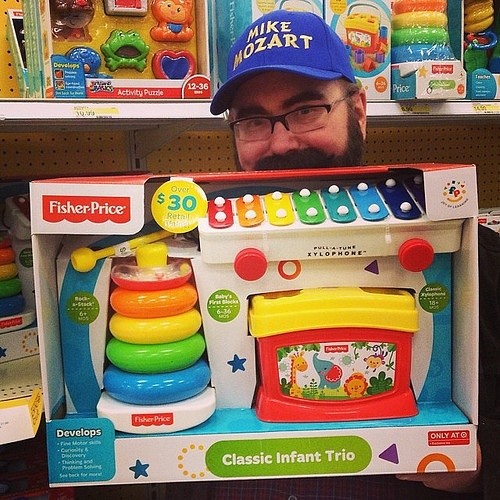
xmin=225 ymin=91 xmax=355 ymax=141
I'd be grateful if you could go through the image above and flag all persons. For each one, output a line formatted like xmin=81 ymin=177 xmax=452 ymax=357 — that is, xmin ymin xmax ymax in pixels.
xmin=164 ymin=7 xmax=500 ymax=500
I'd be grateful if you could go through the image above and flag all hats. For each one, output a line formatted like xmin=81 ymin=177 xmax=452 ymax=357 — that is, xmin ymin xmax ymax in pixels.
xmin=210 ymin=10 xmax=357 ymax=115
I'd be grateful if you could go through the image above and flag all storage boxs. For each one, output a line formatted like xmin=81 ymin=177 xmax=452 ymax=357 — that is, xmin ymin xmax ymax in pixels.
xmin=28 ymin=163 xmax=480 ymax=488
xmin=0 ymin=178 xmax=45 ymax=364
xmin=23 ymin=0 xmax=215 ymax=102
xmin=463 ymin=0 xmax=500 ymax=100
xmin=324 ymin=0 xmax=393 ymax=100
xmin=206 ymin=0 xmax=324 ymax=102
xmin=6 ymin=9 xmax=28 ymax=98
xmin=391 ymin=0 xmax=467 ymax=101
